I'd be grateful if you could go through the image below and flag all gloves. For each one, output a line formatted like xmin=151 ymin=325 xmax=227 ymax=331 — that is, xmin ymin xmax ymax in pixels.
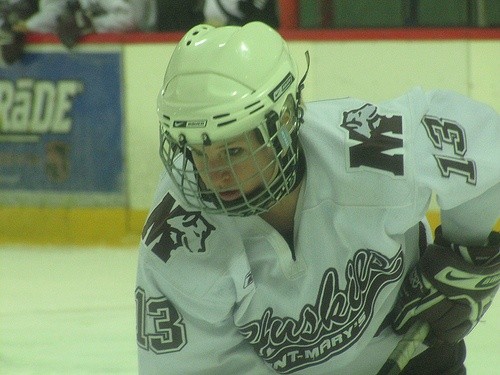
xmin=393 ymin=225 xmax=499 ymax=349
xmin=56 ymin=10 xmax=93 ymax=47
xmin=0 ymin=25 xmax=25 ymax=65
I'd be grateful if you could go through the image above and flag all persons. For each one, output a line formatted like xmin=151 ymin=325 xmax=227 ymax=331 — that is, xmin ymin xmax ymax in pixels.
xmin=134 ymin=19 xmax=499 ymax=374
xmin=0 ymin=0 xmax=280 ymax=65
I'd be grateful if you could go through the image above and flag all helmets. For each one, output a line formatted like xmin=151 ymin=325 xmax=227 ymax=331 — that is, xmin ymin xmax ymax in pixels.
xmin=153 ymin=20 xmax=301 ymax=152
